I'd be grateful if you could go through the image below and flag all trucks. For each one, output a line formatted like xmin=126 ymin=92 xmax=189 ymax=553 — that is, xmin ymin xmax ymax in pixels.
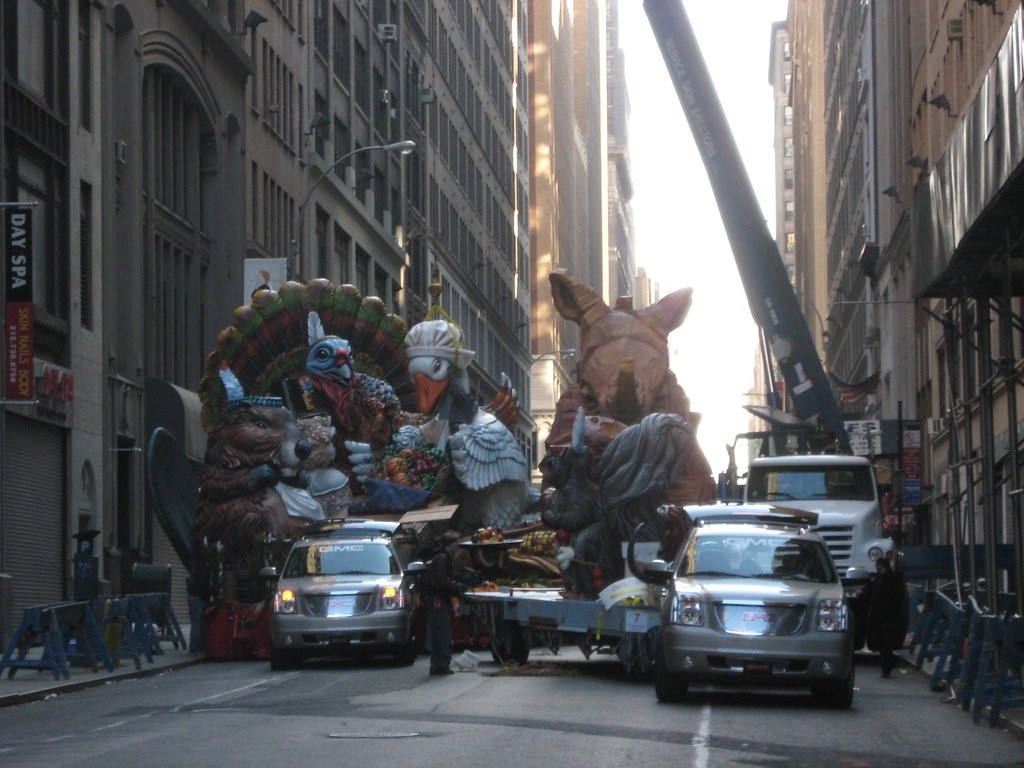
xmin=463 ymin=503 xmax=870 ymax=709
xmin=258 ymin=518 xmax=429 ymax=667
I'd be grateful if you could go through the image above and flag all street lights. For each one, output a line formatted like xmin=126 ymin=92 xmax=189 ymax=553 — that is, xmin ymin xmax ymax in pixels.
xmin=290 ymin=140 xmax=416 ymax=281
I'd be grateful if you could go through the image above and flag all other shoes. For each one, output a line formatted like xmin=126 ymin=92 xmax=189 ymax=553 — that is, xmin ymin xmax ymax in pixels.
xmin=891 ymin=654 xmax=899 ymax=669
xmin=881 ymin=664 xmax=891 ymax=679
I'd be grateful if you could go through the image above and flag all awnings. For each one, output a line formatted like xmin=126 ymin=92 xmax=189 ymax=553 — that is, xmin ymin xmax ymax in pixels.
xmin=918 ymin=158 xmax=1024 ymax=388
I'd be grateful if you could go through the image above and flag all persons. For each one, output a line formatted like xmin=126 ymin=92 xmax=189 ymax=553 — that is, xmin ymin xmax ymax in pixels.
xmin=423 ymin=531 xmax=469 ymax=675
xmin=864 ymin=556 xmax=911 ymax=678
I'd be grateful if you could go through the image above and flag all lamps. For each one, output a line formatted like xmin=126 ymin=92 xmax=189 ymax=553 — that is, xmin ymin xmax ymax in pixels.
xmin=493 ymin=296 xmax=507 ymax=305
xmin=470 ymin=261 xmax=485 ymax=274
xmin=569 ymin=368 xmax=577 ymax=378
xmin=236 ymin=9 xmax=267 ymax=36
xmin=305 ymin=111 xmax=330 ymax=136
xmin=821 ymin=316 xmax=833 ymax=338
xmin=514 ymin=323 xmax=527 ymax=333
xmin=907 ymin=155 xmax=928 ymax=168
xmin=882 ymin=187 xmax=904 ymax=203
xmin=929 ymin=95 xmax=957 ymax=118
xmin=977 ymin=0 xmax=1002 ymax=16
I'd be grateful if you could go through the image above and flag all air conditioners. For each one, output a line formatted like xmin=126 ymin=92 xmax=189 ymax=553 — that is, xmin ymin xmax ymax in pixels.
xmin=380 ymin=25 xmax=399 ymax=42
xmin=378 ymin=90 xmax=389 ymax=103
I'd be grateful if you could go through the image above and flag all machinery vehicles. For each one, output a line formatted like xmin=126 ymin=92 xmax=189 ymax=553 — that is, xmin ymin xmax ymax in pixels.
xmin=641 ymin=0 xmax=910 ymax=650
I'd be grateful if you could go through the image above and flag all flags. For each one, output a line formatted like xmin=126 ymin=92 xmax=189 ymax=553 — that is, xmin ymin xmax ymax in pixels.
xmin=829 ymin=368 xmax=882 ymax=403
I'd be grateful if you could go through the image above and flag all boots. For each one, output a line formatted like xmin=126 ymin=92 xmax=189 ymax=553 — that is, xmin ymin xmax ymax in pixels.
xmin=430 ymin=655 xmax=454 ymax=675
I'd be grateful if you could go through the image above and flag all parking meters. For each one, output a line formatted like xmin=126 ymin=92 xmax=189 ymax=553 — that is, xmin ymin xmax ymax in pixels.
xmin=73 ymin=529 xmax=100 ymax=598
